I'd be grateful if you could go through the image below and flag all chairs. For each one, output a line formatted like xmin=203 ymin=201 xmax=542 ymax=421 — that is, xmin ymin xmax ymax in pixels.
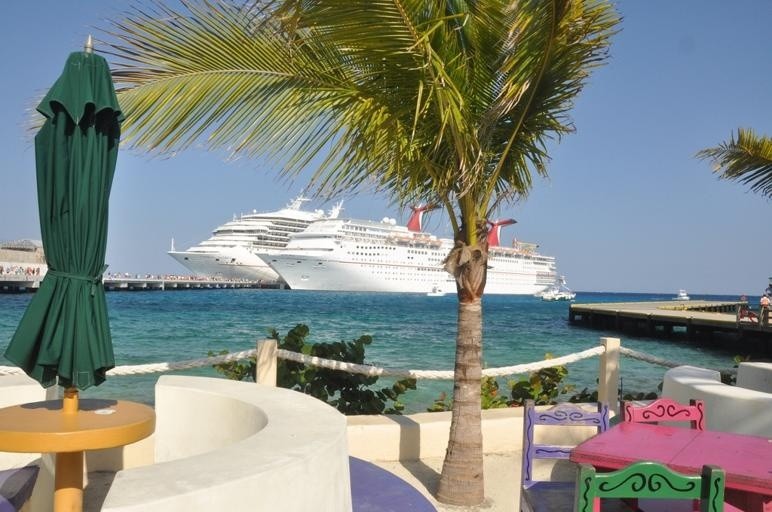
xmin=573 ymin=462 xmax=725 ymax=512
xmin=620 ymin=398 xmax=706 ymax=512
xmin=518 ymin=399 xmax=637 ymax=512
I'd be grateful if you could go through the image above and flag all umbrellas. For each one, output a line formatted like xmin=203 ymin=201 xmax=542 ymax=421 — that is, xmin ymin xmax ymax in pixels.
xmin=0 ymin=34 xmax=125 ymax=412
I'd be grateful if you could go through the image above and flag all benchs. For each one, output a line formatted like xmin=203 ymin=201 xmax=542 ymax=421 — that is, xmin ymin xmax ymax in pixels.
xmin=657 ymin=364 xmax=772 ymax=438
xmin=0 ymin=373 xmax=58 ymax=512
xmin=735 ymin=361 xmax=772 ymax=394
xmin=99 ymin=375 xmax=353 ymax=512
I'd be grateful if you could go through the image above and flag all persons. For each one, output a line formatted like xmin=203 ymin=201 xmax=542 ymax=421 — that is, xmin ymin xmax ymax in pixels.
xmin=759 ymin=294 xmax=770 ymax=307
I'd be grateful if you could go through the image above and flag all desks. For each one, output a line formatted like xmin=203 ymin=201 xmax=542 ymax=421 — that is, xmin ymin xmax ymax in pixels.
xmin=0 ymin=399 xmax=156 ymax=512
xmin=569 ymin=420 xmax=772 ymax=512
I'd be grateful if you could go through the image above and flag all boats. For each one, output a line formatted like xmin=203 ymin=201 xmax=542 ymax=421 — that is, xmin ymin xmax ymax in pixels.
xmin=673 ymin=290 xmax=688 ymax=301
xmin=534 ymin=277 xmax=576 ymax=300
